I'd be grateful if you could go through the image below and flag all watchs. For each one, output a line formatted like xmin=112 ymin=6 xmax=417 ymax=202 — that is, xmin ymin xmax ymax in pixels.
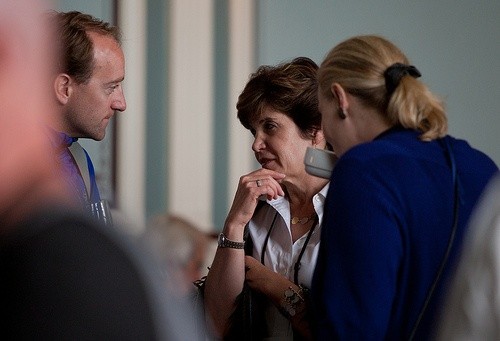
xmin=216 ymin=232 xmax=246 ymax=249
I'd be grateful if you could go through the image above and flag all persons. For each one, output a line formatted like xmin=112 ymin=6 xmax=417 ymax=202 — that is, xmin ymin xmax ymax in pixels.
xmin=196 ymin=56 xmax=333 ymax=341
xmin=305 ymin=35 xmax=500 ymax=341
xmin=24 ymin=10 xmax=128 ymax=226
xmin=438 ymin=177 xmax=500 ymax=341
xmin=0 ymin=0 xmax=160 ymax=341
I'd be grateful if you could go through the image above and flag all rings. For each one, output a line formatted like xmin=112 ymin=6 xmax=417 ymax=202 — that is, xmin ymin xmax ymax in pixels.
xmin=256 ymin=179 xmax=262 ymax=187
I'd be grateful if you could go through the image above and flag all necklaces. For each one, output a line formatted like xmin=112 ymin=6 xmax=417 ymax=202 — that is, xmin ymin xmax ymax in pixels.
xmin=290 ymin=210 xmax=318 ymax=225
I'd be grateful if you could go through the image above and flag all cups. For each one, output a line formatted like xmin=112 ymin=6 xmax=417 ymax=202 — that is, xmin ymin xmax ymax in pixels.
xmin=304 ymin=146 xmax=338 ymax=178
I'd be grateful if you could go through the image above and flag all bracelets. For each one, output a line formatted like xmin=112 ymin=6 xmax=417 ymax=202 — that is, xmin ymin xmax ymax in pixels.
xmin=276 ymin=283 xmax=309 ymax=321
